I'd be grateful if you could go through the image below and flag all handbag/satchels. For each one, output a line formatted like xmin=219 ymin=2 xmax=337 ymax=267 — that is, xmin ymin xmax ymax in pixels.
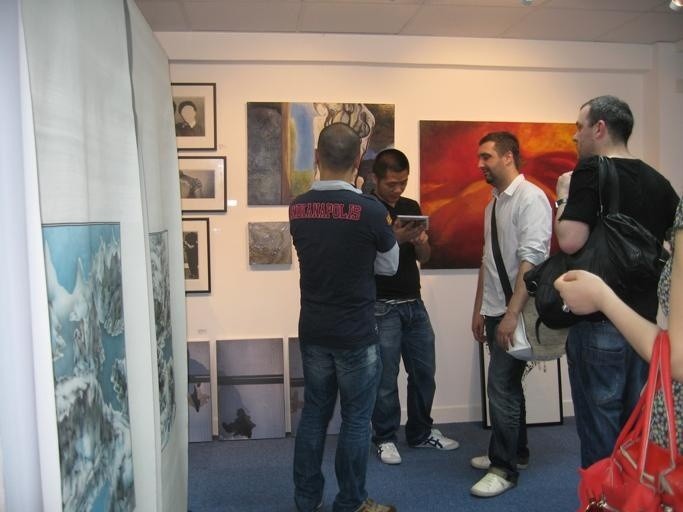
xmin=523 ymin=153 xmax=670 ymax=345
xmin=503 ymin=303 xmax=568 ymax=362
xmin=574 ymin=328 xmax=683 ymax=511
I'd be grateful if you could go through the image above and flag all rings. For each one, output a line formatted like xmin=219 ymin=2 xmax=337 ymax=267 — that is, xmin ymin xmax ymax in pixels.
xmin=562 ymin=303 xmax=569 ymax=312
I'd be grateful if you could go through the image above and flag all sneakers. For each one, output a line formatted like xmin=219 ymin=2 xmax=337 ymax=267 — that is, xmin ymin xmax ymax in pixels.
xmin=470 ymin=455 xmax=529 ymax=471
xmin=409 ymin=428 xmax=460 ymax=451
xmin=470 ymin=471 xmax=517 ymax=498
xmin=354 ymin=497 xmax=396 ymax=511
xmin=376 ymin=441 xmax=402 ymax=465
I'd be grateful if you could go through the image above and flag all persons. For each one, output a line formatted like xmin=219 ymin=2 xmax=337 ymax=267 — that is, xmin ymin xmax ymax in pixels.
xmin=184 ymin=232 xmax=198 ymax=279
xmin=368 ymin=149 xmax=461 ymax=465
xmin=175 ymin=101 xmax=204 ymax=136
xmin=554 ymin=190 xmax=683 ymax=458
xmin=288 ymin=121 xmax=401 ymax=511
xmin=469 ymin=131 xmax=555 ymax=498
xmin=556 ymin=96 xmax=681 ymax=470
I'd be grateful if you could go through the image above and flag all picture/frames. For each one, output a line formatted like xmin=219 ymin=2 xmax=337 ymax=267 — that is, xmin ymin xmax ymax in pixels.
xmin=170 ymin=82 xmax=217 ymax=150
xmin=181 ymin=217 xmax=211 ymax=294
xmin=177 ymin=155 xmax=227 ymax=212
xmin=477 ymin=329 xmax=563 ymax=428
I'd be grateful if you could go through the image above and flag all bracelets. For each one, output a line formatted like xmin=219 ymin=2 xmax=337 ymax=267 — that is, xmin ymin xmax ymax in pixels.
xmin=555 ymin=198 xmax=568 ymax=208
